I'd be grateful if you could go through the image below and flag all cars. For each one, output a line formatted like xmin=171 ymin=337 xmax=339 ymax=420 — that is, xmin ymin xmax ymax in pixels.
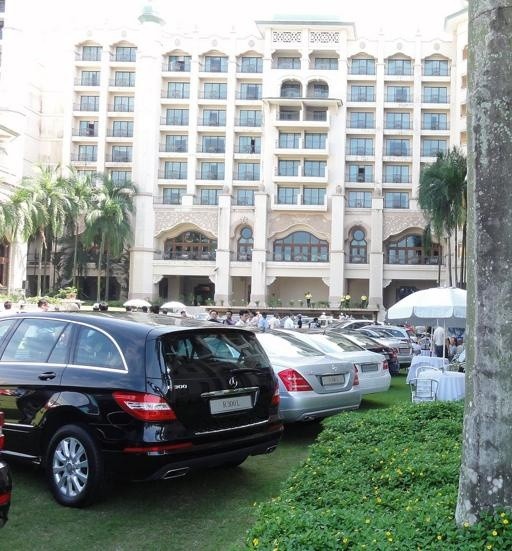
xmin=295 ymin=317 xmax=432 ymax=395
xmin=241 ymin=327 xmax=364 ymax=422
xmin=0 ymin=409 xmax=11 ymax=525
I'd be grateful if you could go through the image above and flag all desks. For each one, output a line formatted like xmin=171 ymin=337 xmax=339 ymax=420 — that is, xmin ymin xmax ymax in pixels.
xmin=423 ymin=370 xmax=466 ymax=405
xmin=403 ymin=356 xmax=449 ymax=386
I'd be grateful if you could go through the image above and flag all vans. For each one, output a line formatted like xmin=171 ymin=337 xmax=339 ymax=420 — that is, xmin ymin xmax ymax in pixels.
xmin=0 ymin=310 xmax=284 ymax=507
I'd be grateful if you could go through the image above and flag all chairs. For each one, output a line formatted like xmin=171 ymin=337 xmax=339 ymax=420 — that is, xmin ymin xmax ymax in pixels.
xmin=415 ymin=365 xmax=440 ymax=380
xmin=409 ymin=377 xmax=440 ymax=402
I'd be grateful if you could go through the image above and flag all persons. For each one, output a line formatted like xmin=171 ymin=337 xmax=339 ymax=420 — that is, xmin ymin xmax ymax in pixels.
xmin=432 ymin=326 xmax=465 ymax=373
xmin=346 ymin=292 xmax=351 ymax=307
xmin=360 ymin=292 xmax=367 ymax=308
xmin=303 ymin=293 xmax=315 ymax=307
xmin=391 ymin=345 xmax=400 ymax=374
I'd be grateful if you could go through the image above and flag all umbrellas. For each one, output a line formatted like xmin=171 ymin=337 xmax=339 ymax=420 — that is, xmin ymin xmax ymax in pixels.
xmin=384 ymin=285 xmax=467 ymax=371
